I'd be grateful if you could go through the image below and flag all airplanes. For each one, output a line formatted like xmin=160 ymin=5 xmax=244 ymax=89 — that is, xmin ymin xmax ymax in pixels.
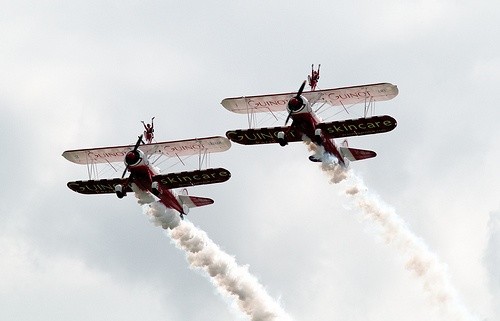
xmin=61 ymin=116 xmax=232 ymax=220
xmin=221 ymin=63 xmax=399 ymax=169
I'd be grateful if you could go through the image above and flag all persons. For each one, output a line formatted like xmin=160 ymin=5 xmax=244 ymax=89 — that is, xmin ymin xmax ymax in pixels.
xmin=141 ymin=117 xmax=155 ymax=143
xmin=308 ymin=64 xmax=321 ymax=91
